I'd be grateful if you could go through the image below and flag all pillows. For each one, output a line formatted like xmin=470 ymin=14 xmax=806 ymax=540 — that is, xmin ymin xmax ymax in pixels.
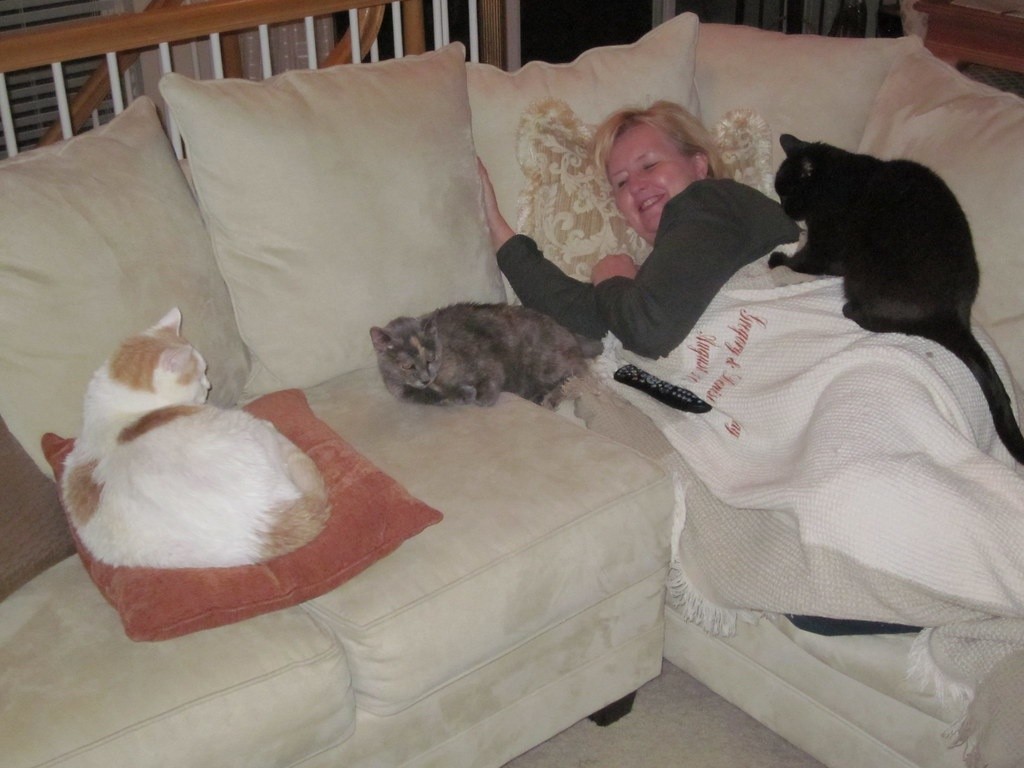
xmin=463 ymin=9 xmax=701 ymax=310
xmin=158 ymin=41 xmax=505 ymax=396
xmin=0 ymin=414 xmax=78 ymax=601
xmin=0 ymin=92 xmax=251 ymax=481
xmin=859 ymin=32 xmax=1024 ymax=325
xmin=42 ymin=387 xmax=443 ymax=641
xmin=516 ymin=96 xmax=773 ymax=285
xmin=693 ymin=22 xmax=906 ymax=205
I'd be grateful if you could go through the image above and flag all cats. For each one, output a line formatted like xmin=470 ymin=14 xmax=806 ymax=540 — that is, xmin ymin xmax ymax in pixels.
xmin=58 ymin=302 xmax=334 ymax=573
xmin=765 ymin=130 xmax=1024 ymax=468
xmin=369 ymin=299 xmax=607 ymax=413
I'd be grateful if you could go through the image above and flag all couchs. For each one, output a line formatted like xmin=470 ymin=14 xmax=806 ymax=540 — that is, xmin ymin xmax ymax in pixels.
xmin=0 ymin=10 xmax=1024 ymax=768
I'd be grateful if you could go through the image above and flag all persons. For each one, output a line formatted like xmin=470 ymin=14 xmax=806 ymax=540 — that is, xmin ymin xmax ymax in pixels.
xmin=476 ymin=98 xmax=1024 ymax=613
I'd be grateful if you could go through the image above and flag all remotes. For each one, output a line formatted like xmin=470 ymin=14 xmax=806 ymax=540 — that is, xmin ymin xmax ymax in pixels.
xmin=614 ymin=364 xmax=712 ymax=414
